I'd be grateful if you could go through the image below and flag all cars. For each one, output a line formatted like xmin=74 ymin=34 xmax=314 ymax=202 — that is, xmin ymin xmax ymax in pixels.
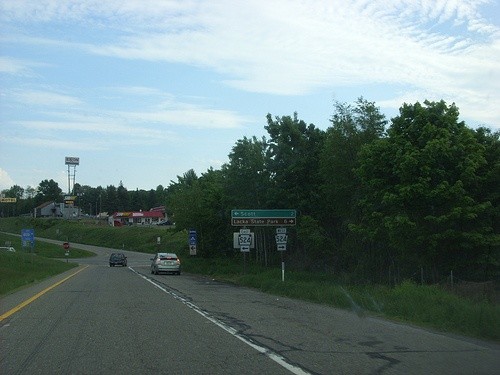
xmin=0 ymin=246 xmax=16 ymax=253
xmin=109 ymin=253 xmax=128 ymax=267
xmin=150 ymin=252 xmax=181 ymax=275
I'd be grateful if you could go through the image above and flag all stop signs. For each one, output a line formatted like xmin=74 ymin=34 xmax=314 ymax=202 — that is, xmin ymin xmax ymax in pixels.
xmin=63 ymin=243 xmax=69 ymax=248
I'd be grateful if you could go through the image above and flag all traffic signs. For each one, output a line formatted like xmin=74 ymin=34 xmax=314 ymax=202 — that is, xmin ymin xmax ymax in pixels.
xmin=238 ymin=234 xmax=253 ymax=252
xmin=231 ymin=210 xmax=297 ymax=227
xmin=275 ymin=233 xmax=289 ymax=251
